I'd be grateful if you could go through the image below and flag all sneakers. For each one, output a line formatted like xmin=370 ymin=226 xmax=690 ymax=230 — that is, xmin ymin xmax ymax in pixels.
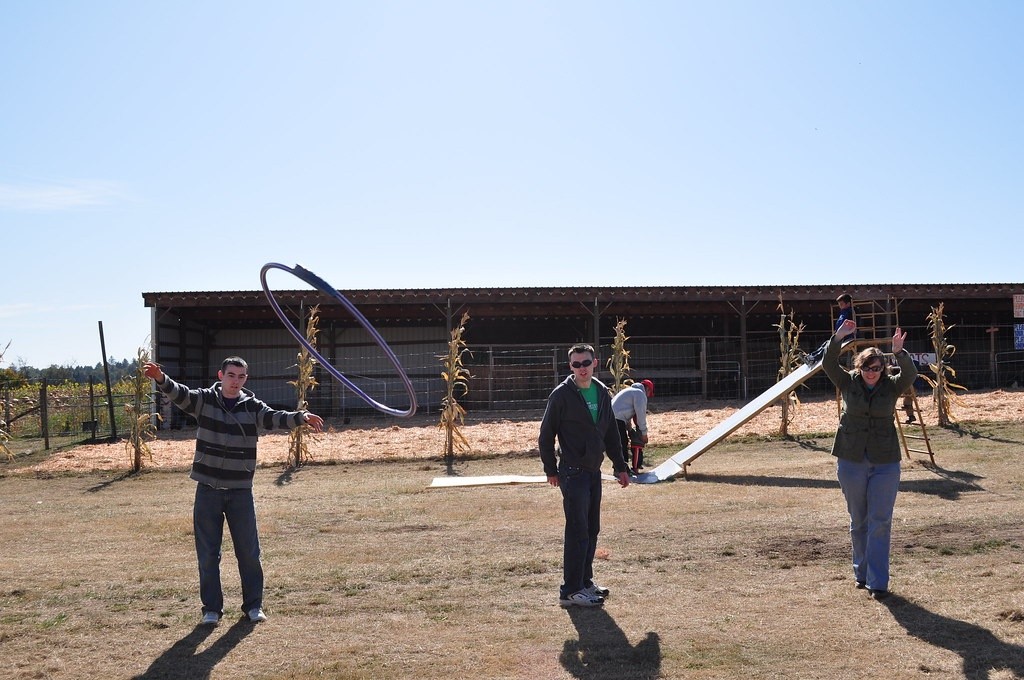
xmin=559 ymin=588 xmax=605 ymax=606
xmin=202 ymin=611 xmax=219 ymax=625
xmin=585 ymin=584 xmax=609 ymax=596
xmin=248 ymin=607 xmax=267 ymax=622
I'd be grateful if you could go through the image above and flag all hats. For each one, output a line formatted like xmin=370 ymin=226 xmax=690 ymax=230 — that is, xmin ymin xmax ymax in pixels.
xmin=643 ymin=380 xmax=654 ymax=398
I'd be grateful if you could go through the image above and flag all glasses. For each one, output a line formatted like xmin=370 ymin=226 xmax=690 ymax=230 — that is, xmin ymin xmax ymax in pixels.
xmin=571 ymin=359 xmax=592 ymax=368
xmin=861 ymin=366 xmax=881 ymax=372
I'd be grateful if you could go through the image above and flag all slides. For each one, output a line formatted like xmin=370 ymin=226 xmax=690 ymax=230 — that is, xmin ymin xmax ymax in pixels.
xmin=639 ymin=339 xmax=894 ymax=482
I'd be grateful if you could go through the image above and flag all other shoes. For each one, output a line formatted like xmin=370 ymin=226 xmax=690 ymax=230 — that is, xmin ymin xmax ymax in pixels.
xmin=799 ymin=351 xmax=811 ymax=363
xmin=804 ymin=356 xmax=817 ymax=369
xmin=634 ymin=471 xmax=638 ymax=475
xmin=639 ymin=466 xmax=643 ymax=469
xmin=906 ymin=415 xmax=916 ymax=424
xmin=868 ymin=589 xmax=888 ymax=599
xmin=855 ymin=581 xmax=866 ymax=589
xmin=902 ymin=405 xmax=913 ymax=410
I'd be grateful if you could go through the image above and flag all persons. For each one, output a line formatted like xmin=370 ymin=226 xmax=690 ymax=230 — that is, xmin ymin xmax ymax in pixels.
xmin=802 ymin=293 xmax=854 ymax=368
xmin=822 ymin=319 xmax=918 ymax=600
xmin=611 ymin=379 xmax=654 ymax=474
xmin=538 ymin=343 xmax=628 ymax=607
xmin=142 ymin=357 xmax=323 ymax=625
xmin=886 ymin=350 xmax=916 ymax=424
